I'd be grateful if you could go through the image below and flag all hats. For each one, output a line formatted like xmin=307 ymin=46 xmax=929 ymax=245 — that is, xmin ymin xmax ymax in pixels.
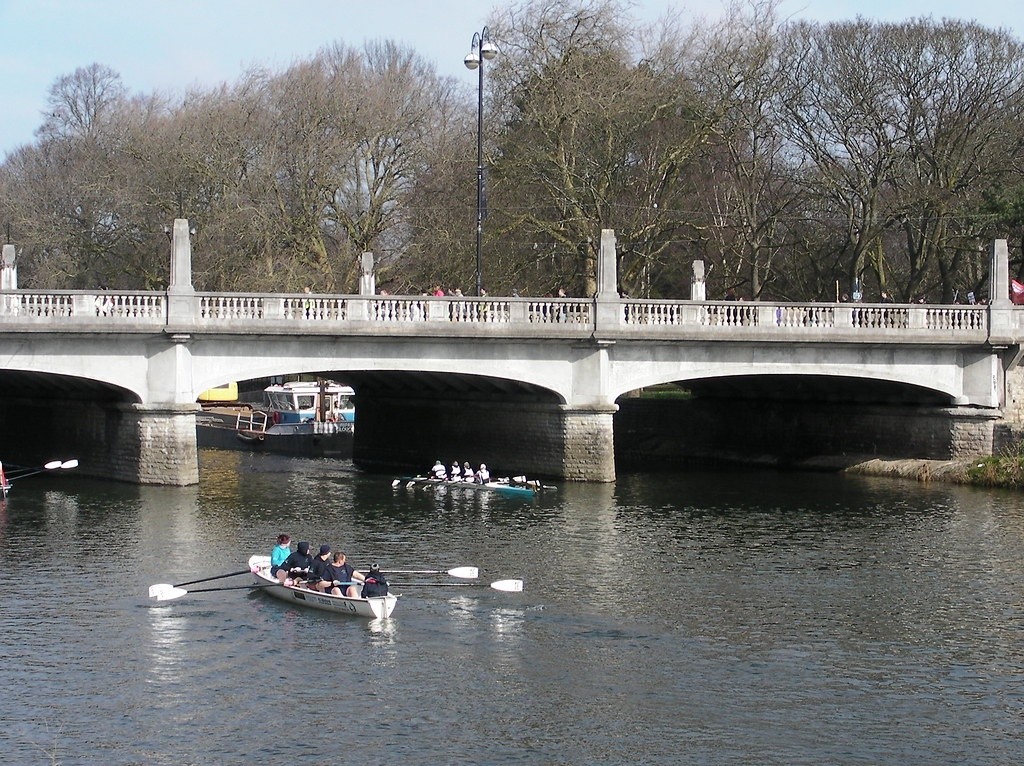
xmin=320 ymin=544 xmax=330 ymax=555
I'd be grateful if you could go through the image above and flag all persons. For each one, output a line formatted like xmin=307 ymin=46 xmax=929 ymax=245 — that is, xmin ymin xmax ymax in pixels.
xmin=460 ymin=462 xmax=474 ymax=483
xmin=446 ymin=461 xmax=462 ymax=483
xmin=722 ymin=287 xmax=736 ymax=323
xmin=361 ymin=563 xmax=387 ymax=598
xmin=281 ymin=542 xmax=313 ymax=589
xmin=475 ymin=464 xmax=489 ymax=485
xmin=317 ymin=551 xmax=365 ymax=598
xmin=97 ymin=288 xmax=117 ymax=316
xmin=305 ymin=287 xmax=312 ymax=319
xmin=837 ymin=289 xmax=893 ymax=303
xmin=952 ymin=297 xmax=988 ymax=327
xmin=432 ymin=281 xmax=489 ymax=322
xmin=803 ymin=298 xmax=818 ymax=325
xmin=380 ymin=290 xmax=397 ymax=320
xmin=308 ymin=544 xmax=331 ymax=593
xmin=543 ymin=288 xmax=567 ymax=323
xmin=270 ymin=534 xmax=291 ymax=583
xmin=427 ymin=460 xmax=447 ymax=479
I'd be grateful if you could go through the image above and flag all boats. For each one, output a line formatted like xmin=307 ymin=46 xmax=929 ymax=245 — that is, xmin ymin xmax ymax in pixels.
xmin=195 ymin=381 xmax=355 ymax=458
xmin=248 ymin=554 xmax=397 ymax=619
xmin=392 ymin=475 xmax=535 ymax=497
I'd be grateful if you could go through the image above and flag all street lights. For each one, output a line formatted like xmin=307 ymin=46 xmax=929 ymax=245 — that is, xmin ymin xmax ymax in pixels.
xmin=463 ymin=24 xmax=499 ymax=297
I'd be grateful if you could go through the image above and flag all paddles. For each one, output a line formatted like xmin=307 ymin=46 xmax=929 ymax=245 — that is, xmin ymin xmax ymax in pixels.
xmin=358 ymin=566 xmax=478 ymax=578
xmin=338 ymin=579 xmax=523 ymax=592
xmin=422 ymin=476 xmax=448 ymax=491
xmin=156 ymin=579 xmax=323 ymax=601
xmin=5 ymin=459 xmax=78 ymax=481
xmin=405 ymin=477 xmax=428 ymax=487
xmin=392 ymin=472 xmax=430 ymax=486
xmin=149 ymin=565 xmax=273 ymax=598
xmin=4 ymin=461 xmax=62 ymax=474
xmin=437 ymin=474 xmax=474 ymax=492
xmin=491 ymin=475 xmax=558 ymax=490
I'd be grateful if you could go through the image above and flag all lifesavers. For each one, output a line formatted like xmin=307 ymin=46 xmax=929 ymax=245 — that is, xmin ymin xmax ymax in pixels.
xmin=272 ymin=411 xmax=279 ymax=425
